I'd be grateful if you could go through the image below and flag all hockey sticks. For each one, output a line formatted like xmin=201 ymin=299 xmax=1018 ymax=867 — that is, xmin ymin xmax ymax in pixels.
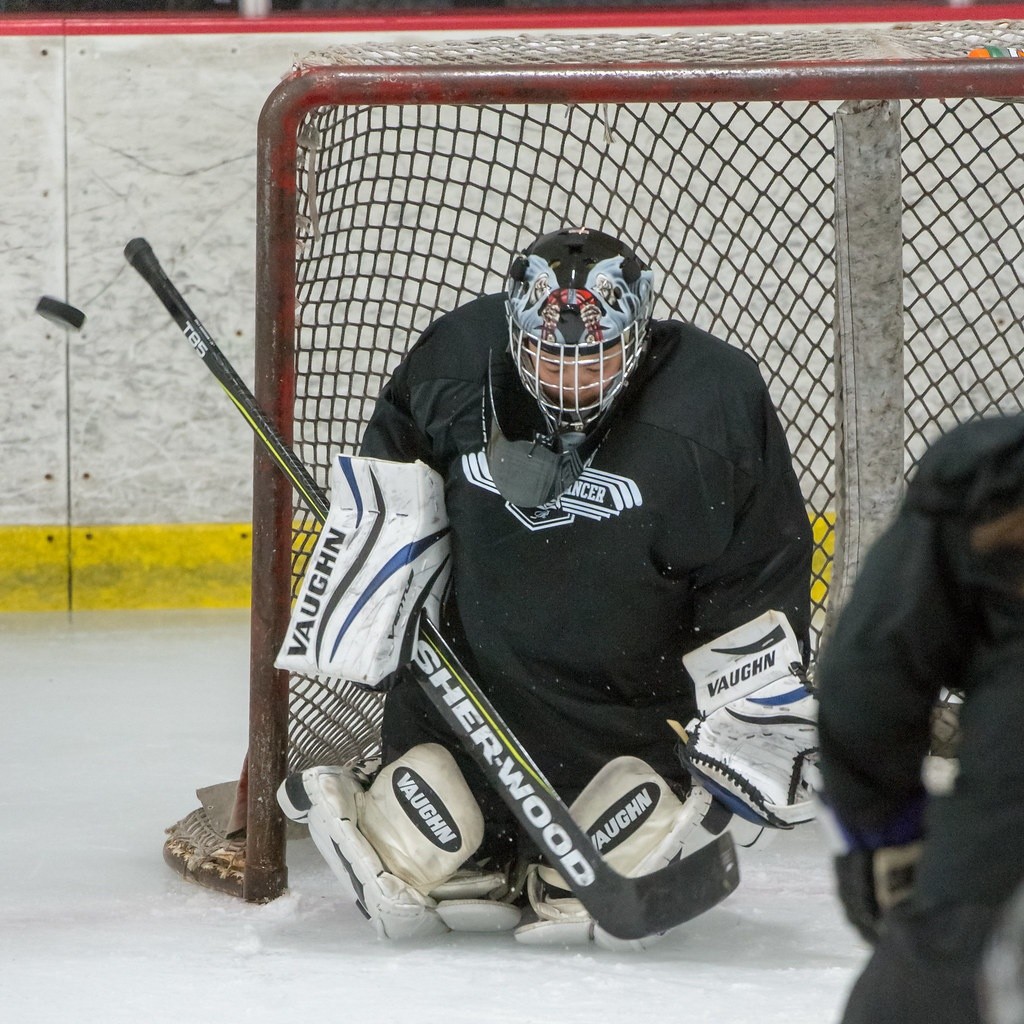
xmin=125 ymin=236 xmax=739 ymax=939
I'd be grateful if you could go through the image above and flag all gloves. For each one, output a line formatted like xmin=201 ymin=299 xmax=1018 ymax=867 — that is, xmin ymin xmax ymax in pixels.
xmin=815 ymin=795 xmax=928 ymax=950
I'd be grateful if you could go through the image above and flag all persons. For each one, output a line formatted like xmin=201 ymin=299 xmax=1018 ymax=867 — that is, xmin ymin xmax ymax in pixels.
xmin=816 ymin=412 xmax=1023 ymax=1024
xmin=272 ymin=226 xmax=818 ymax=950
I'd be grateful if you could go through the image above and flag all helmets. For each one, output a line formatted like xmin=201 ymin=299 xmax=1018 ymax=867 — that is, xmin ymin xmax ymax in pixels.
xmin=504 ymin=226 xmax=654 ymax=444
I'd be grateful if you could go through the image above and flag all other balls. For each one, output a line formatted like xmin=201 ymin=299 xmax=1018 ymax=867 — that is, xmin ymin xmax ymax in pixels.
xmin=35 ymin=296 xmax=86 ymax=331
